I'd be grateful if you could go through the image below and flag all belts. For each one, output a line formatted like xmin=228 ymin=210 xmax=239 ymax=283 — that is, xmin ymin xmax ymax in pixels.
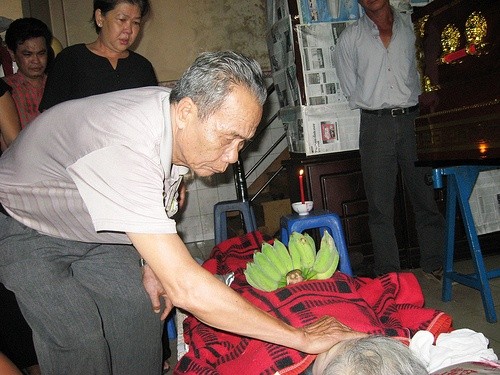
xmin=362 ymin=103 xmax=420 ymax=118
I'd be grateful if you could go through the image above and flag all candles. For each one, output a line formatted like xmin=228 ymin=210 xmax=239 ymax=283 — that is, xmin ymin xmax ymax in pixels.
xmin=298 ymin=169 xmax=305 ymax=204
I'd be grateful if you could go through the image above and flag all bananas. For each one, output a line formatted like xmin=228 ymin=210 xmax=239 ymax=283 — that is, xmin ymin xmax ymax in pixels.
xmin=243 ymin=230 xmax=340 ymax=292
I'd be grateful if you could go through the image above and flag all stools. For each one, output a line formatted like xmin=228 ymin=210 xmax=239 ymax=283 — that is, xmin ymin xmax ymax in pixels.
xmin=214 ymin=199 xmax=258 ymax=246
xmin=279 ymin=209 xmax=354 ymax=279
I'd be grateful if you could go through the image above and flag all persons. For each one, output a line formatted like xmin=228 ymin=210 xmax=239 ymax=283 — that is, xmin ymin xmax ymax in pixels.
xmin=330 ymin=0 xmax=457 ymax=293
xmin=1 ymin=16 xmax=58 ymax=159
xmin=0 ymin=75 xmax=44 ymax=375
xmin=0 ymin=46 xmax=375 ymax=375
xmin=39 ymin=0 xmax=194 ymax=375
xmin=209 ymin=221 xmax=434 ymax=375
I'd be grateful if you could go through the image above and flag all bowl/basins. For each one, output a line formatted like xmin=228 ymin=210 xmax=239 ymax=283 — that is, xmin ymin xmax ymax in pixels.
xmin=291 ymin=201 xmax=313 ymax=216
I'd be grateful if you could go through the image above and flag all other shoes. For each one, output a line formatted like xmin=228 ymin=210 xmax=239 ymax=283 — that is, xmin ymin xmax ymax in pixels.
xmin=423 ymin=266 xmax=458 ymax=286
